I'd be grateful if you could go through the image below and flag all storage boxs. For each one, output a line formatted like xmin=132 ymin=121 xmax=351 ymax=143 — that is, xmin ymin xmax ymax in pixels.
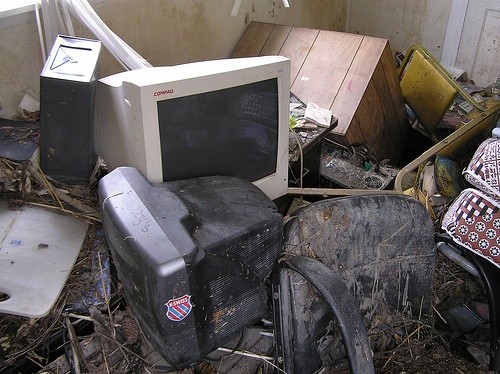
xmin=231 ymin=21 xmax=408 ymax=168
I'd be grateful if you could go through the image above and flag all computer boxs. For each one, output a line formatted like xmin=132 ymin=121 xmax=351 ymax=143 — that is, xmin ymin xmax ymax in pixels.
xmin=37 ymin=34 xmax=103 ymax=185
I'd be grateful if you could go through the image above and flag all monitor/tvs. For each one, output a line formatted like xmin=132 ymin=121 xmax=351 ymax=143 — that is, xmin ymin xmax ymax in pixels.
xmin=97 ymin=166 xmax=286 ymax=371
xmin=94 ymin=57 xmax=293 ymax=204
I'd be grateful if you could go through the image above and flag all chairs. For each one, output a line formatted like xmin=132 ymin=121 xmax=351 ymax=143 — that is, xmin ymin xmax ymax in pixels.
xmin=394 ymin=44 xmax=500 ymax=192
xmin=277 ymin=193 xmax=500 ymax=374
